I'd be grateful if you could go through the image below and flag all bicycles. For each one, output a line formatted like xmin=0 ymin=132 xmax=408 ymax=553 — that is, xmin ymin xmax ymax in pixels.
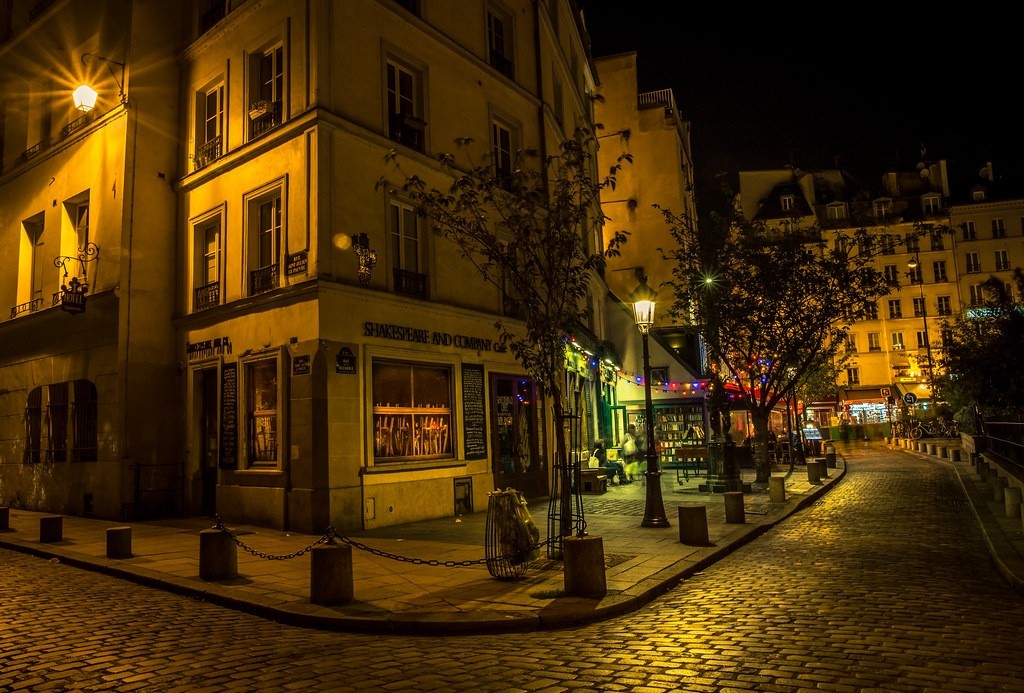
xmin=909 ymin=420 xmax=963 ymax=440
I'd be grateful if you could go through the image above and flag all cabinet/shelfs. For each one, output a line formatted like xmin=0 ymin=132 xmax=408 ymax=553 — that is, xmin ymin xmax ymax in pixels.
xmin=654 ymin=404 xmax=707 ymax=467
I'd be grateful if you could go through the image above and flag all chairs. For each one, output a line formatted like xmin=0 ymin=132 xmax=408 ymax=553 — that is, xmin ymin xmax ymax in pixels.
xmin=768 ymin=441 xmax=778 ymax=464
xmin=576 ymin=460 xmax=608 ymax=495
xmin=781 ymin=442 xmax=792 ymax=464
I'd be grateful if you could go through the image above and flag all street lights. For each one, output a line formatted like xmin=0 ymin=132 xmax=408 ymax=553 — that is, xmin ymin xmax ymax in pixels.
xmin=907 ymin=246 xmax=936 ymax=407
xmin=627 ymin=284 xmax=671 ymax=528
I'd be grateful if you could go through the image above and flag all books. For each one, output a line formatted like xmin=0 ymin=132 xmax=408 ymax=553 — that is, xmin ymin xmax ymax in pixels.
xmin=653 ymin=407 xmax=706 ymax=463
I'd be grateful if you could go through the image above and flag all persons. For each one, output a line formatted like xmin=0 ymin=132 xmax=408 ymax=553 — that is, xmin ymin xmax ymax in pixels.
xmin=621 ymin=424 xmax=647 ymax=482
xmin=592 ymin=439 xmax=632 ymax=484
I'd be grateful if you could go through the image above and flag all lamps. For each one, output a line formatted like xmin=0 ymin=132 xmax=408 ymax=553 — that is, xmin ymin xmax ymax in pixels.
xmin=72 ymin=53 xmax=125 ymax=113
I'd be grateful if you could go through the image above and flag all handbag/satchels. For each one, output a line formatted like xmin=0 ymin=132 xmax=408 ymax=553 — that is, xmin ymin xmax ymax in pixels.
xmin=589 ymin=449 xmax=600 ymax=468
xmin=623 ymin=433 xmax=637 ymax=456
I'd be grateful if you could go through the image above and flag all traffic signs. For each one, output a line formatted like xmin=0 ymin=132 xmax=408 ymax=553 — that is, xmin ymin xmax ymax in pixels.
xmin=904 ymin=392 xmax=917 ymax=405
